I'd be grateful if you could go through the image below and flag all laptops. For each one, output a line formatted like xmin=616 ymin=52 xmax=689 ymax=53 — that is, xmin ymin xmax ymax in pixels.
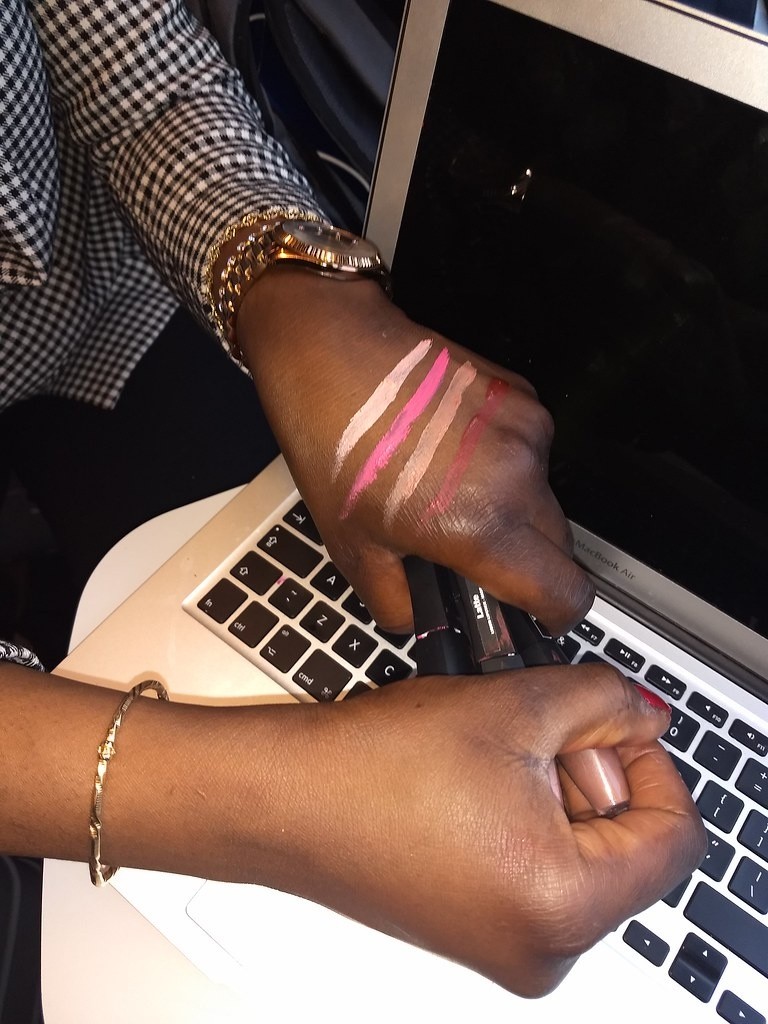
xmin=38 ymin=0 xmax=768 ymax=1024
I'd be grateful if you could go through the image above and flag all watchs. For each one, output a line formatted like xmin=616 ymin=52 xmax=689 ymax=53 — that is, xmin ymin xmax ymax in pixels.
xmin=206 ymin=220 xmax=401 ymax=376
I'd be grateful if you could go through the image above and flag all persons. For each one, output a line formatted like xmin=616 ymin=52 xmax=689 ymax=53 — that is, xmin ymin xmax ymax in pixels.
xmin=0 ymin=0 xmax=707 ymax=1024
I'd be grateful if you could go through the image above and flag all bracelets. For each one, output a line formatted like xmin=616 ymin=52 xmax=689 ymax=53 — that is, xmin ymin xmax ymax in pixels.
xmin=83 ymin=682 xmax=168 ymax=886
xmin=202 ymin=204 xmax=328 ymax=338
